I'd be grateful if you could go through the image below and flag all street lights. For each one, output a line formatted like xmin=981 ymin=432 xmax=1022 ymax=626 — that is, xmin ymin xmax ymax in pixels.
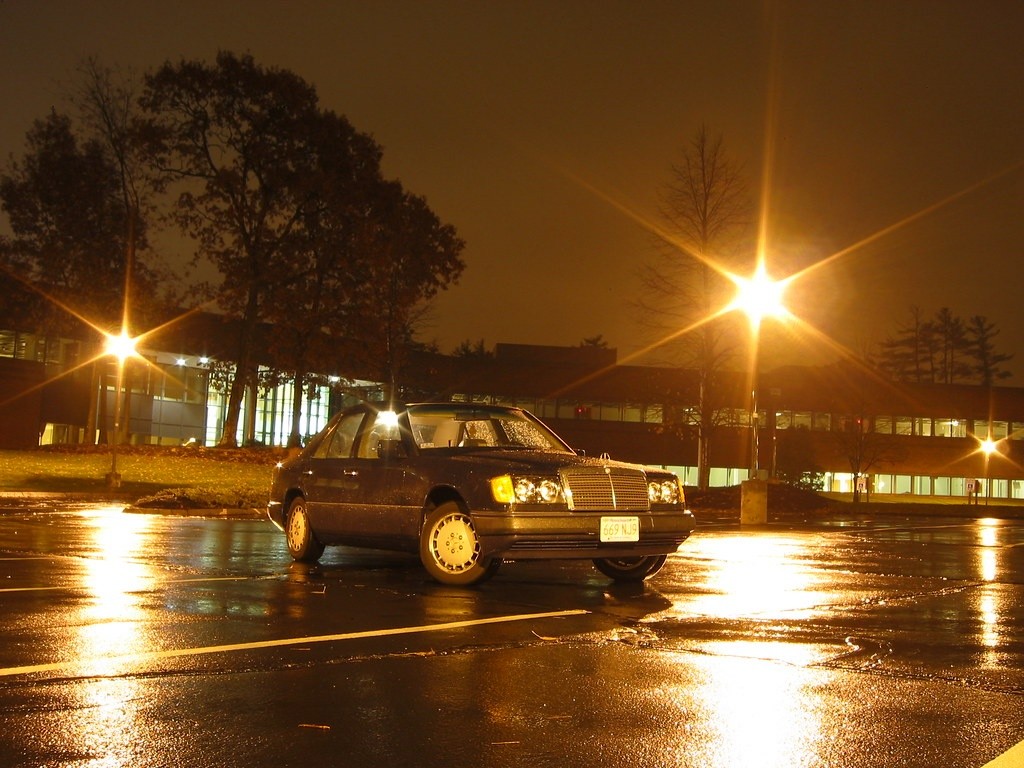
xmin=107 ymin=332 xmax=135 ymax=489
xmin=736 ymin=272 xmax=781 ymax=526
xmin=981 ymin=439 xmax=996 ymax=515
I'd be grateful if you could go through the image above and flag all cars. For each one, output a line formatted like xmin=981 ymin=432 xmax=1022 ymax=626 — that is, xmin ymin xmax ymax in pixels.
xmin=269 ymin=399 xmax=696 ymax=584
